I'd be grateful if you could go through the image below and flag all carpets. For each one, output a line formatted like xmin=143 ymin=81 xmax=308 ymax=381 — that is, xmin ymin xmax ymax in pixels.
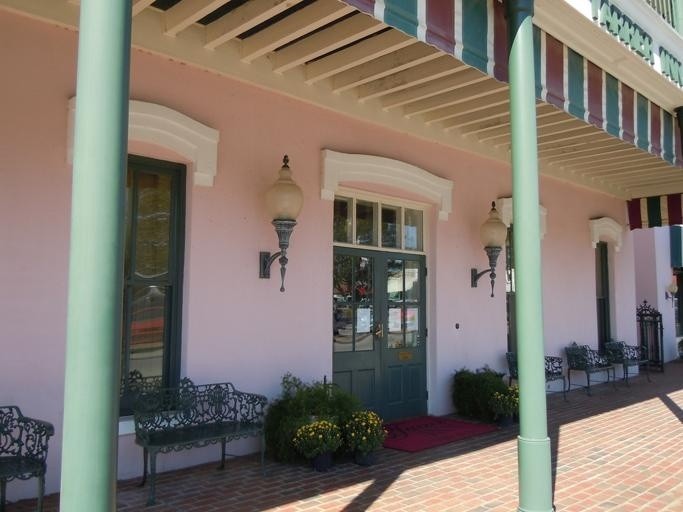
xmin=381 ymin=415 xmax=497 ymax=452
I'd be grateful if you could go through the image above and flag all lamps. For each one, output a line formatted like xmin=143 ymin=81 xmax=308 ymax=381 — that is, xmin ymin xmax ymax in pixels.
xmin=665 ymin=280 xmax=678 ymax=307
xmin=259 ymin=154 xmax=304 ymax=292
xmin=471 ymin=201 xmax=508 ymax=298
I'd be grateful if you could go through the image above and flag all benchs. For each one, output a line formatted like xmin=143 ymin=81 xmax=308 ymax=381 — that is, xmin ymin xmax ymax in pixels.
xmin=506 ymin=352 xmax=568 ymax=402
xmin=0 ymin=405 xmax=55 ymax=512
xmin=133 ymin=376 xmax=268 ymax=507
xmin=605 ymin=340 xmax=653 ymax=387
xmin=565 ymin=344 xmax=618 ymax=396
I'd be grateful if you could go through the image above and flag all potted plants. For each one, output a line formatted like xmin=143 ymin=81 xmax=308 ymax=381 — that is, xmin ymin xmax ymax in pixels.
xmin=264 ymin=370 xmax=366 ymax=463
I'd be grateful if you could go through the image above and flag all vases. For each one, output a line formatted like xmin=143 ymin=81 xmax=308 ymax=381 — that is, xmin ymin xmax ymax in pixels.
xmin=513 ymin=411 xmax=519 ymax=424
xmin=496 ymin=412 xmax=513 ymax=426
xmin=312 ymin=452 xmax=332 ymax=472
xmin=354 ymin=448 xmax=375 ymax=466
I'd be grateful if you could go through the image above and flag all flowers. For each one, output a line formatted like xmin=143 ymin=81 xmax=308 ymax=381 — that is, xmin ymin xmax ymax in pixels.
xmin=290 ymin=420 xmax=344 ymax=456
xmin=508 ymin=383 xmax=518 ymax=397
xmin=342 ymin=407 xmax=389 ymax=450
xmin=488 ymin=391 xmax=520 ymax=417
